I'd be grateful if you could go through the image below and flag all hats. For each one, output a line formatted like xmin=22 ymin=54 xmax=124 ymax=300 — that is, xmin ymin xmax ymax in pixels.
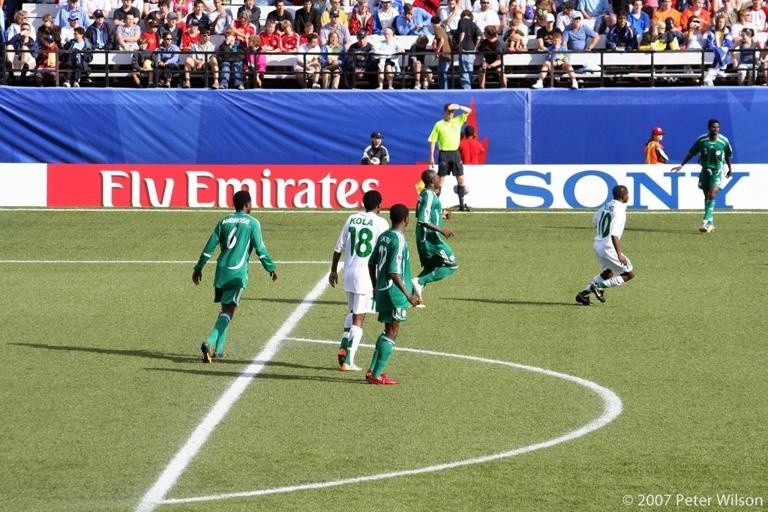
xmin=546 ymin=12 xmax=581 ymax=21
xmin=168 ymin=12 xmax=178 ymax=21
xmin=652 ymin=128 xmax=664 ymax=135
xmin=357 ymin=30 xmax=366 ymax=38
xmin=68 ymin=9 xmax=103 ymax=20
xmin=371 ymin=131 xmax=384 ymax=138
xmin=330 ymin=11 xmax=339 ymax=16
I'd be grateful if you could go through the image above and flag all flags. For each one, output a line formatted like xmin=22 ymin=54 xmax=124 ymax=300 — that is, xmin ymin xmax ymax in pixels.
xmin=465 ymin=97 xmax=479 ymax=141
xmin=479 ymin=137 xmax=488 ymax=164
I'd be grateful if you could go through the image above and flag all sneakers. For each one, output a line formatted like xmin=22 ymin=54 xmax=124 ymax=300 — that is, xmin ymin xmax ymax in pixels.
xmin=576 ymin=284 xmax=605 ymax=306
xmin=338 ymin=348 xmax=362 ymax=371
xmin=149 ymin=81 xmax=191 ymax=88
xmin=412 ymin=277 xmax=423 ymax=299
xmin=532 ymin=82 xmax=543 ymax=89
xmin=571 ymin=82 xmax=578 ymax=89
xmin=211 ymin=83 xmax=245 ymax=90
xmin=699 ymin=220 xmax=715 ymax=233
xmin=367 ymin=370 xmax=396 ymax=384
xmin=202 ymin=341 xmax=224 ymax=363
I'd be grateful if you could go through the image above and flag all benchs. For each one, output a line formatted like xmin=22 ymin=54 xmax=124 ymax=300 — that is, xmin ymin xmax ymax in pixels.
xmin=1 ymin=0 xmax=768 ymax=87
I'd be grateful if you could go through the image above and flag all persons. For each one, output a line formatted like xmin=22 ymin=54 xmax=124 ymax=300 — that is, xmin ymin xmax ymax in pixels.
xmin=328 ymin=191 xmax=390 ymax=371
xmin=365 ymin=204 xmax=420 ymax=385
xmin=575 ymin=185 xmax=637 ymax=306
xmin=460 ymin=125 xmax=486 ymax=165
xmin=194 ymin=190 xmax=277 ymax=363
xmin=428 ymin=102 xmax=472 ymax=211
xmin=643 ymin=126 xmax=669 ymax=163
xmin=0 ymin=0 xmax=768 ymax=95
xmin=360 ymin=131 xmax=389 ymax=164
xmin=672 ymin=118 xmax=735 ymax=234
xmin=411 ymin=168 xmax=460 ymax=307
